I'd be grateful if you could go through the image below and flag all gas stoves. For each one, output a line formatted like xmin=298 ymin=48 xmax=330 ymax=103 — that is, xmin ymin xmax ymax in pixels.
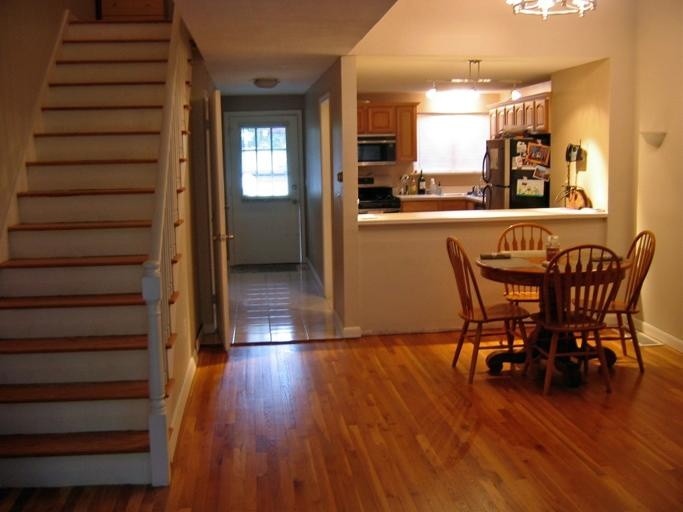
xmin=358 ymin=186 xmax=401 ymax=208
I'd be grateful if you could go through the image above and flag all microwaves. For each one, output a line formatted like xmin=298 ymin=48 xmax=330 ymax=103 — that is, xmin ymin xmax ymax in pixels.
xmin=357 ymin=133 xmax=398 ymax=166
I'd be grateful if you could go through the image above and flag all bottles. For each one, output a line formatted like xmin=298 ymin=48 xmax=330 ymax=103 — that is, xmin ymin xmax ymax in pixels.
xmin=409 ymin=170 xmax=442 ymax=196
xmin=546 ymin=234 xmax=561 ymax=264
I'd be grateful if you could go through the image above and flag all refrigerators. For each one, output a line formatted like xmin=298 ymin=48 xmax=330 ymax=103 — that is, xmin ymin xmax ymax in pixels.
xmin=481 ymin=137 xmax=550 ymax=209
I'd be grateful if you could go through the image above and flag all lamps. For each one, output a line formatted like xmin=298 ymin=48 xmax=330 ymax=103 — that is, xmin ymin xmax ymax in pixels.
xmin=638 ymin=130 xmax=667 ymax=149
xmin=250 ymin=76 xmax=282 ymax=90
xmin=505 ymin=1 xmax=600 ymax=24
xmin=419 ymin=57 xmax=524 ymax=102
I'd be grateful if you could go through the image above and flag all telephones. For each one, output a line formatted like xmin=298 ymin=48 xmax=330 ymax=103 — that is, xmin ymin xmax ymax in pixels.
xmin=566 ymin=143 xmax=583 ymax=162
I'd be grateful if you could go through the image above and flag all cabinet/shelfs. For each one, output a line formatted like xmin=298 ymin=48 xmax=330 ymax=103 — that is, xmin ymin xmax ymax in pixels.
xmin=398 ymin=197 xmax=476 ymax=213
xmin=353 ymin=101 xmax=396 ymax=133
xmin=485 ymin=91 xmax=551 ymax=143
xmin=393 ymin=101 xmax=422 ymax=163
